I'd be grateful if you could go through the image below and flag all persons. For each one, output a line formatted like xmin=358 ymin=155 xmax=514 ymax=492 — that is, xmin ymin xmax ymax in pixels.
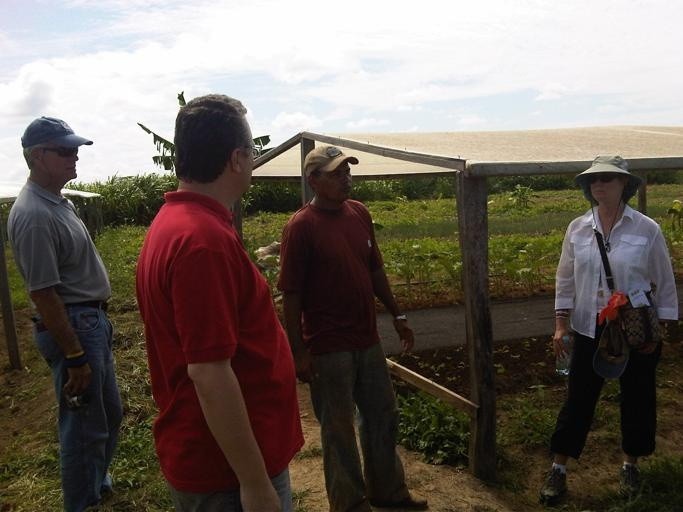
xmin=276 ymin=146 xmax=429 ymax=512
xmin=137 ymin=95 xmax=305 ymax=511
xmin=6 ymin=116 xmax=124 ymax=511
xmin=541 ymin=156 xmax=679 ymax=505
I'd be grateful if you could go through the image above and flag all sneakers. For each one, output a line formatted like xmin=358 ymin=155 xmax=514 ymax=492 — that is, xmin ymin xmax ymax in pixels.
xmin=619 ymin=466 xmax=639 ymax=494
xmin=538 ymin=466 xmax=567 ymax=502
xmin=372 ymin=490 xmax=428 ymax=511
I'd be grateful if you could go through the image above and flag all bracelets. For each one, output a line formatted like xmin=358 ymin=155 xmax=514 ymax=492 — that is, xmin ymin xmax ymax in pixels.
xmin=64 ymin=354 xmax=88 ymax=368
xmin=65 ymin=350 xmax=86 ymax=359
xmin=395 ymin=314 xmax=408 ymax=322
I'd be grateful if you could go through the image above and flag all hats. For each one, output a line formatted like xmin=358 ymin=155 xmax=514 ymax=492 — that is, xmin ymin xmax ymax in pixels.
xmin=21 ymin=116 xmax=94 ymax=151
xmin=575 ymin=154 xmax=641 ymax=202
xmin=591 ymin=321 xmax=629 ymax=381
xmin=304 ymin=145 xmax=358 ymax=178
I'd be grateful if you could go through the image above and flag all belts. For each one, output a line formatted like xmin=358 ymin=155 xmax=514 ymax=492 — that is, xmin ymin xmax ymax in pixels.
xmin=65 ymin=301 xmax=109 ymax=313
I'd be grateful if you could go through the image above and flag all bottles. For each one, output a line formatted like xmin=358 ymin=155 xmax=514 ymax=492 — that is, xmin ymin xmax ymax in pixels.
xmin=555 ymin=334 xmax=574 ymax=377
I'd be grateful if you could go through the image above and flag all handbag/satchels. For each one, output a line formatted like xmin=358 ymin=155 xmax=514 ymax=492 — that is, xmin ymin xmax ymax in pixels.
xmin=621 ymin=291 xmax=664 ymax=344
